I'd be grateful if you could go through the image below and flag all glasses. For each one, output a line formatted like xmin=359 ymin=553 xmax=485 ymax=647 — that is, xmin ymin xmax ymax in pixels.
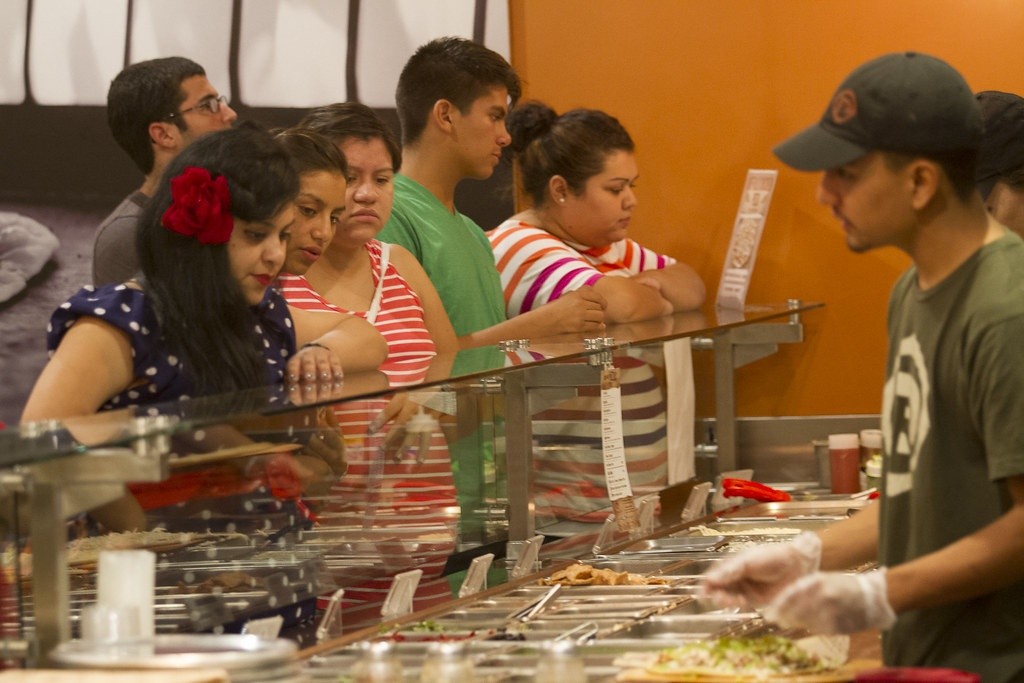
xmin=161 ymin=96 xmax=230 ymax=122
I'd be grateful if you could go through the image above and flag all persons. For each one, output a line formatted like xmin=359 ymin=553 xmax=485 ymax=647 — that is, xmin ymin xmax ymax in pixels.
xmin=697 ymin=50 xmax=1022 ymax=683
xmin=374 ymin=37 xmax=607 ymax=552
xmin=489 ymin=100 xmax=706 ymax=528
xmin=19 ymin=58 xmax=461 ymax=644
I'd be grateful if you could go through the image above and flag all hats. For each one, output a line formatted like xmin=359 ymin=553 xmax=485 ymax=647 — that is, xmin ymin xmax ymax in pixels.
xmin=973 ymin=91 xmax=1024 ymax=182
xmin=773 ymin=52 xmax=984 ymax=172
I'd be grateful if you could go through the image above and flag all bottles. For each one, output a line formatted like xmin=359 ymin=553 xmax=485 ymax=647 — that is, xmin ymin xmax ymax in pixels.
xmin=866 ymin=456 xmax=883 ymax=490
xmin=403 ymin=406 xmax=442 ymax=460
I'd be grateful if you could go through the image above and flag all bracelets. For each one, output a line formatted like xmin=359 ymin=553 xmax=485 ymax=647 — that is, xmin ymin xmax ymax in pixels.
xmin=301 ymin=343 xmax=332 ymax=352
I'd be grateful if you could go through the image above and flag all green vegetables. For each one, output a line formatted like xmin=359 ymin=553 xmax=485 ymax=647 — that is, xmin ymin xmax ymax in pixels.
xmin=656 ymin=638 xmax=821 ymax=671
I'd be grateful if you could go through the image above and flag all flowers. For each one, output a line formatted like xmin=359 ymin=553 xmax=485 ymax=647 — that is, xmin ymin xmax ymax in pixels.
xmin=162 ymin=167 xmax=235 ymax=245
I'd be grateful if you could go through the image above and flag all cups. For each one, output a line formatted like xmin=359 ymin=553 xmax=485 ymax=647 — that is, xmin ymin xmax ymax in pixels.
xmin=861 ymin=428 xmax=883 ymax=471
xmin=827 ymin=433 xmax=861 ymax=494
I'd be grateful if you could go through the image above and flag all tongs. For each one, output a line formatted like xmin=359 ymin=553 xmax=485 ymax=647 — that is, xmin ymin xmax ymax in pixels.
xmin=507 ymin=584 xmax=563 ymax=620
